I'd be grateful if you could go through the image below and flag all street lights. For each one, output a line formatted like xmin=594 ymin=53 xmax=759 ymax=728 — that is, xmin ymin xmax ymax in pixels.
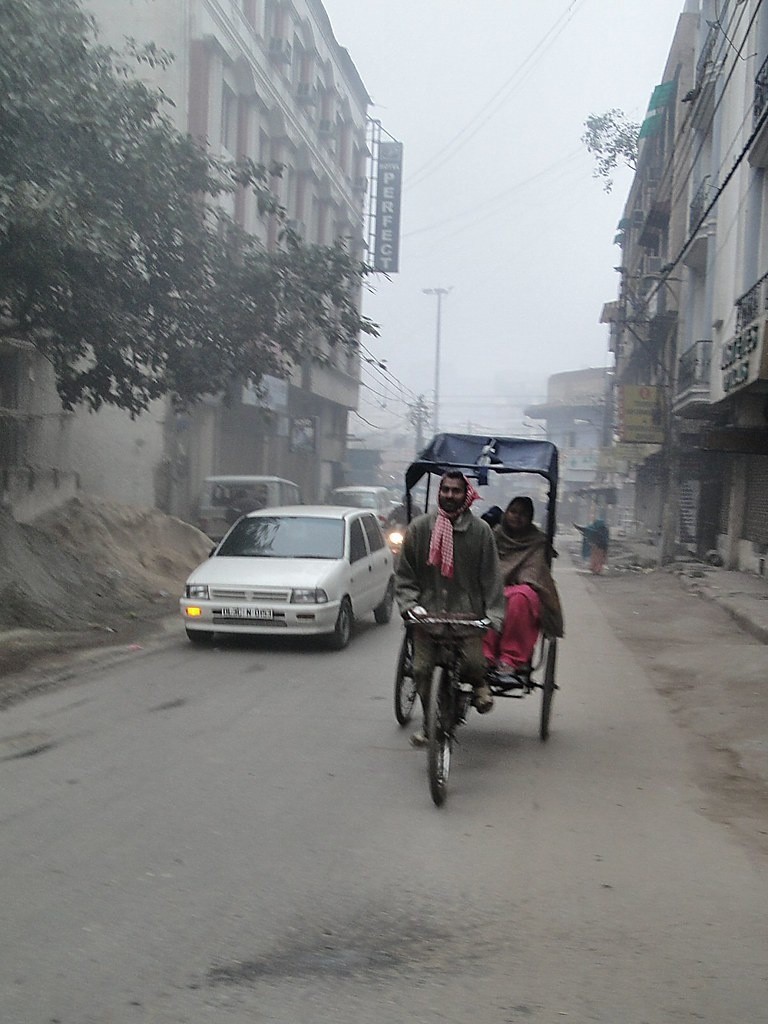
xmin=424 ymin=283 xmax=451 ymax=436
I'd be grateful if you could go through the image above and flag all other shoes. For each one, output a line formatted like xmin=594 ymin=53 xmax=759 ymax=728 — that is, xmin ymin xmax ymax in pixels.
xmin=409 ymin=731 xmax=425 ymax=746
xmin=475 ymin=687 xmax=492 ymax=713
xmin=495 ymin=662 xmax=514 ymax=675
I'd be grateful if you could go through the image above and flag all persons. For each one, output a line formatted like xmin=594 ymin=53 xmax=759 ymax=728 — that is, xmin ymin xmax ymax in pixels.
xmin=394 ymin=470 xmax=504 ymax=748
xmin=571 ymin=516 xmax=610 ymax=575
xmin=477 ymin=496 xmax=566 ymax=692
xmin=382 ymin=493 xmax=421 ymax=534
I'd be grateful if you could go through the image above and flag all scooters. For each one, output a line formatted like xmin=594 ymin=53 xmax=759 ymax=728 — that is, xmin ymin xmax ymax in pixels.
xmin=379 ymin=515 xmax=408 ymax=564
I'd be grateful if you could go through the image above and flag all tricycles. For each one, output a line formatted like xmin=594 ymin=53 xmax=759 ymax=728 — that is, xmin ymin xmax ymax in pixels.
xmin=391 ymin=433 xmax=561 ymax=806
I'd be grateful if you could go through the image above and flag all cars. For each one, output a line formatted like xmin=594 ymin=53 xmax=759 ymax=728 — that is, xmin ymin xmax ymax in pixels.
xmin=179 ymin=506 xmax=393 ymax=647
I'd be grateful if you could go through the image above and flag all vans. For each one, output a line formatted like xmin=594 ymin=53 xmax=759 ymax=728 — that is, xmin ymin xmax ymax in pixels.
xmin=327 ymin=487 xmax=393 ymax=530
xmin=198 ymin=475 xmax=303 ymax=543
